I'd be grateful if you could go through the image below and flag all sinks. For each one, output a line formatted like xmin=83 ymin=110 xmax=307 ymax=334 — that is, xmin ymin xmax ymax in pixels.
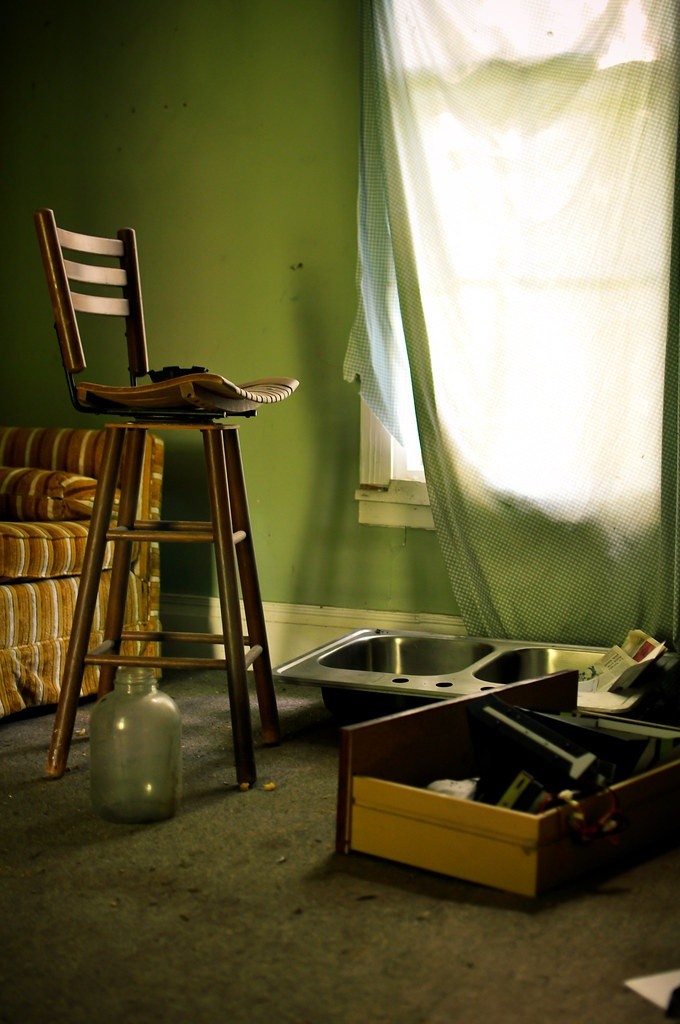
xmin=272 ymin=626 xmax=505 ymax=729
xmin=464 ymin=640 xmax=680 ymax=715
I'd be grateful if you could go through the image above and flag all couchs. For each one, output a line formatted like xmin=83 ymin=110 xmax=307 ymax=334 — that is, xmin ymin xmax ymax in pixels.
xmin=1 ymin=423 xmax=165 ymax=717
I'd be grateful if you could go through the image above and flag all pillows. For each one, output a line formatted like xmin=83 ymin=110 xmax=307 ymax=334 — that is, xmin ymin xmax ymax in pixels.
xmin=0 ymin=466 xmax=123 ymax=518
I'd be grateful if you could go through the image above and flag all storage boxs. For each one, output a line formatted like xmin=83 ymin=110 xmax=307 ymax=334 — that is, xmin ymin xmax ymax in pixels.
xmin=338 ymin=669 xmax=680 ymax=898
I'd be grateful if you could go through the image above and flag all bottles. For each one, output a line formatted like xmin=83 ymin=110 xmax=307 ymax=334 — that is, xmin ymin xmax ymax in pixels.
xmin=88 ymin=667 xmax=182 ymax=825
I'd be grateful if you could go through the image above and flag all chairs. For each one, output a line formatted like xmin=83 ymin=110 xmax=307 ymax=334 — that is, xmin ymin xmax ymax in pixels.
xmin=35 ymin=208 xmax=298 ymax=790
